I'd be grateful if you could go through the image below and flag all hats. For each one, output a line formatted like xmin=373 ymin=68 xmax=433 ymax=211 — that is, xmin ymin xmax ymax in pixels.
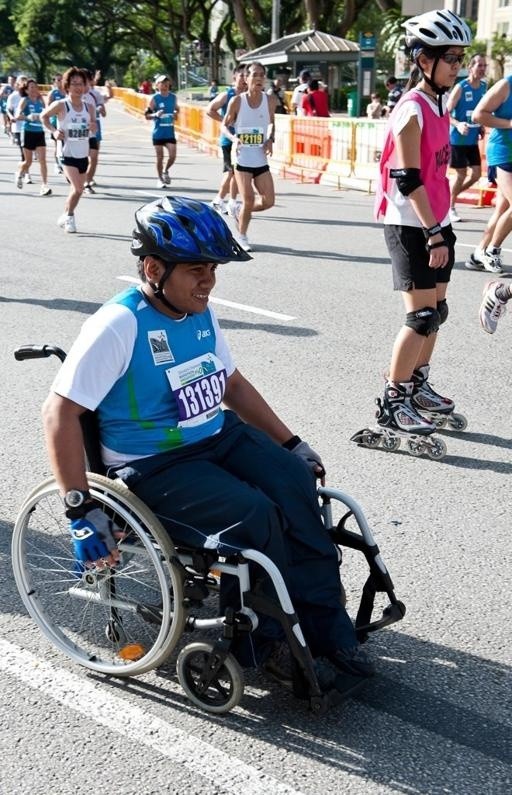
xmin=155 ymin=75 xmax=171 ymax=85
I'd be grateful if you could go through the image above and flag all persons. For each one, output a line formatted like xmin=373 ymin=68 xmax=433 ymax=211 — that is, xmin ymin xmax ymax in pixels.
xmin=137 ymin=73 xmax=179 ymax=189
xmin=366 ymin=54 xmax=511 ymax=274
xmin=478 ymin=278 xmax=512 ymax=336
xmin=1 ymin=66 xmax=117 ymax=232
xmin=207 ymin=61 xmax=332 ymax=253
xmin=375 ymin=7 xmax=479 ymax=438
xmin=38 ymin=196 xmax=378 ymax=704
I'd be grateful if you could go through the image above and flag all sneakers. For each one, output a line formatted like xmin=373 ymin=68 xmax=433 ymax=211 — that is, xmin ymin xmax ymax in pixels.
xmin=449 ymin=207 xmax=462 ymax=222
xmin=479 ymin=280 xmax=508 ymax=334
xmin=13 ymin=161 xmax=101 ymax=197
xmin=158 ymin=170 xmax=170 ymax=189
xmin=210 ymin=196 xmax=252 ymax=252
xmin=320 ymin=642 xmax=375 ymax=676
xmin=259 ymin=639 xmax=335 ymax=687
xmin=59 ymin=212 xmax=76 ymax=232
xmin=465 ymin=247 xmax=505 ymax=273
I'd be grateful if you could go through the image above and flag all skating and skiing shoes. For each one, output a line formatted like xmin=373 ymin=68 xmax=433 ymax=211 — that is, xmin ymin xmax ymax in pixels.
xmin=350 ymin=365 xmax=467 ymax=461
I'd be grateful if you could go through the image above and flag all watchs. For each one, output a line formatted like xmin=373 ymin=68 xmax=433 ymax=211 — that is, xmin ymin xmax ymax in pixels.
xmin=62 ymin=488 xmax=91 ymax=508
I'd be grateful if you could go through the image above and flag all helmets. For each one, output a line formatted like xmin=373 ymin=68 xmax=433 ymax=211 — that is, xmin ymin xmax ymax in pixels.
xmin=401 ymin=9 xmax=472 ymax=48
xmin=131 ymin=195 xmax=253 ymax=264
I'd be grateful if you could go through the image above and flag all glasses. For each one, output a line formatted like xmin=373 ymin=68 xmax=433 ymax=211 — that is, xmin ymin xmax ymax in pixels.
xmin=442 ymin=53 xmax=465 ymax=64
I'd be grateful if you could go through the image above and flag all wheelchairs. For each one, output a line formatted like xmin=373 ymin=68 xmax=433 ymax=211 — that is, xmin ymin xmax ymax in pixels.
xmin=8 ymin=339 xmax=407 ymax=715
